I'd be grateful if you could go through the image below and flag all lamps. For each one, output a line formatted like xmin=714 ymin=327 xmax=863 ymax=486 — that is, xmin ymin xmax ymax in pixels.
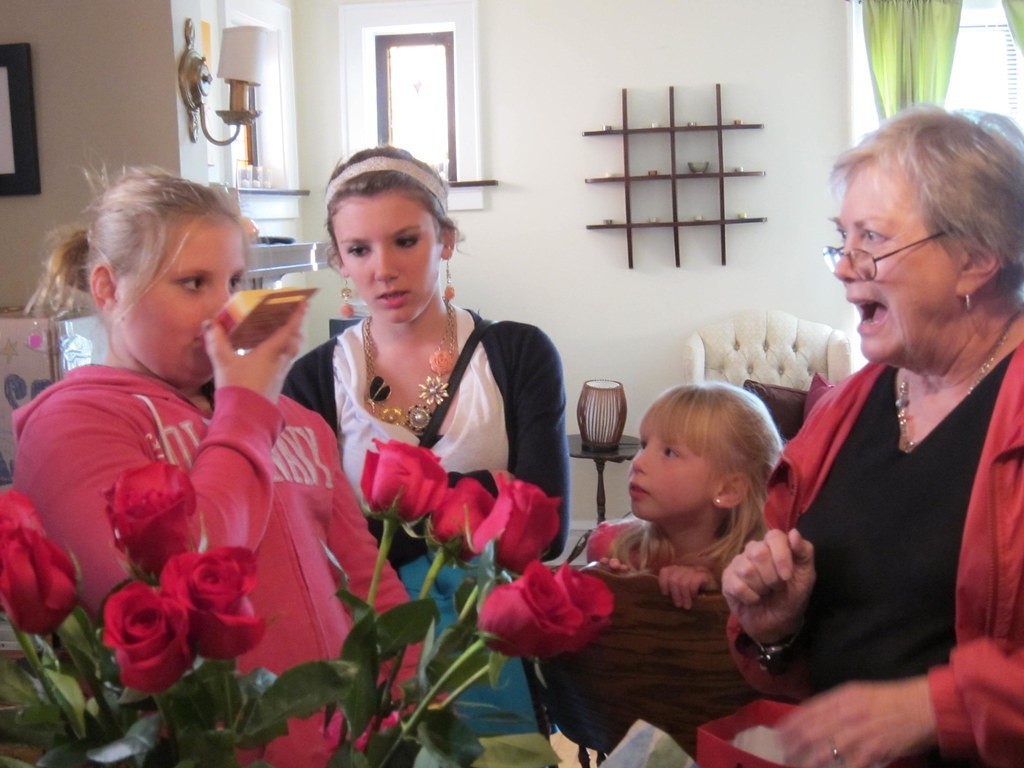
xmin=178 ymin=16 xmax=270 ymax=148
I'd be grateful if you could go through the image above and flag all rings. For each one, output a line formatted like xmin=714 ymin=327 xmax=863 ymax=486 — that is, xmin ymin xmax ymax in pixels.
xmin=829 ymin=736 xmax=845 ymax=765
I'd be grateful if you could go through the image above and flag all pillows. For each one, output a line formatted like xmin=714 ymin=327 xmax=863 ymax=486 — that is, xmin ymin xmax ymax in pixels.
xmin=802 ymin=372 xmax=835 ymax=426
xmin=744 ymin=376 xmax=805 ymax=441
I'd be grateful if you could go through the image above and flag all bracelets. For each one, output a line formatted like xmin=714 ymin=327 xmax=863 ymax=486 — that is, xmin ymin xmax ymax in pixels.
xmin=749 ymin=615 xmax=806 ymax=672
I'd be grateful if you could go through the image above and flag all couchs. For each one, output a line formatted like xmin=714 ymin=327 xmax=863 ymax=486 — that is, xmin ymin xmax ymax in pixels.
xmin=679 ymin=307 xmax=852 ymax=397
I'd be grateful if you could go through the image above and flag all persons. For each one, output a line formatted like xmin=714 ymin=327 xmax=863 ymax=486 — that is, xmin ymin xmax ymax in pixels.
xmin=722 ymin=104 xmax=1024 ymax=768
xmin=584 ymin=380 xmax=783 ymax=608
xmin=9 ymin=168 xmax=435 ymax=766
xmin=278 ymin=144 xmax=571 ymax=738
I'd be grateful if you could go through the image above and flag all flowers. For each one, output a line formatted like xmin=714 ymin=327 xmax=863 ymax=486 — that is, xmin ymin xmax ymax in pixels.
xmin=0 ymin=437 xmax=616 ymax=768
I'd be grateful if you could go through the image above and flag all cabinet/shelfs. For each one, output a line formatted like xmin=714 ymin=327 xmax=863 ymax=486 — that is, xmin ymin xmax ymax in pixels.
xmin=568 ymin=432 xmax=642 ymax=524
xmin=581 ymin=123 xmax=768 ymax=229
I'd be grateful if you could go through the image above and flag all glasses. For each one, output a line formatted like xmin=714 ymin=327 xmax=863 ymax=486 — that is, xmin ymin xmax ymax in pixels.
xmin=822 ymin=229 xmax=948 ymax=280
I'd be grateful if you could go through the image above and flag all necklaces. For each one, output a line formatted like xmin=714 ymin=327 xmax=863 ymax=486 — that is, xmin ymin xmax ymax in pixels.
xmin=894 ymin=303 xmax=1024 ymax=455
xmin=364 ymin=296 xmax=457 ymax=434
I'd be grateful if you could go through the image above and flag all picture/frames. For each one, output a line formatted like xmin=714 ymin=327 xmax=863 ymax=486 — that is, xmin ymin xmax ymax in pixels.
xmin=0 ymin=41 xmax=42 ymax=198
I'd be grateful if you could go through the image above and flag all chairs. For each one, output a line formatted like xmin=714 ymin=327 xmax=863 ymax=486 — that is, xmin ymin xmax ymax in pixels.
xmin=539 ymin=559 xmax=757 ymax=768
